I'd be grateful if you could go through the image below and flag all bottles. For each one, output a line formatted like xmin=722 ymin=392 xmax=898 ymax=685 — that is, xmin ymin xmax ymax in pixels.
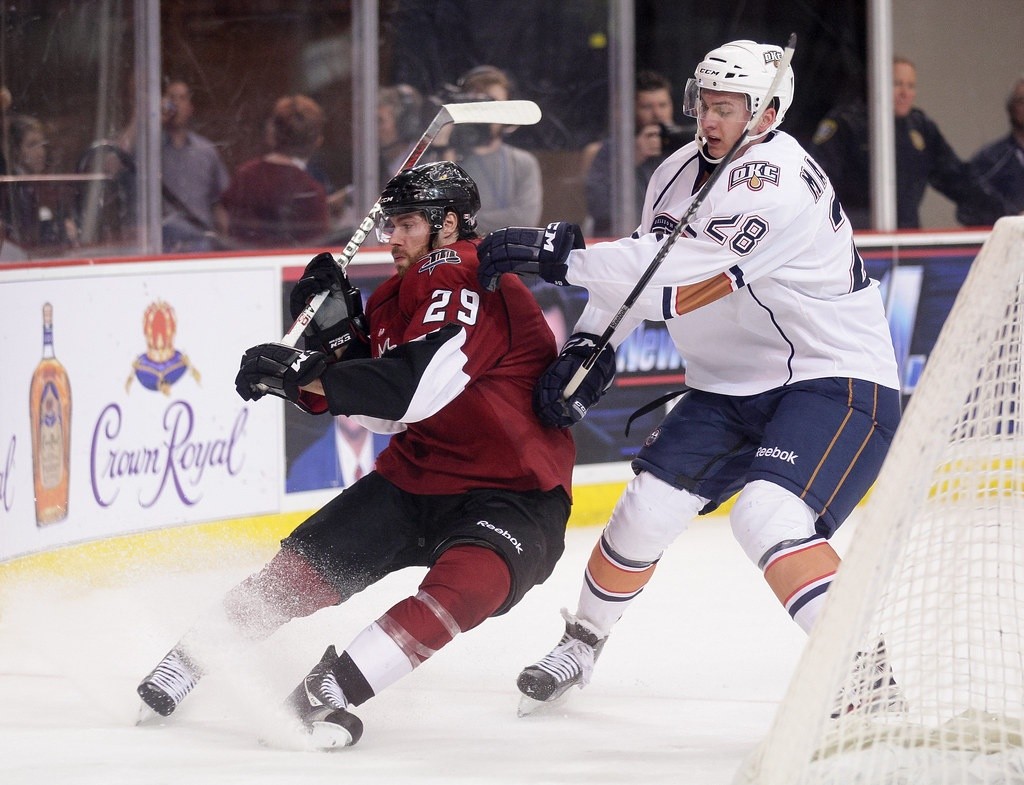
xmin=29 ymin=300 xmax=73 ymax=525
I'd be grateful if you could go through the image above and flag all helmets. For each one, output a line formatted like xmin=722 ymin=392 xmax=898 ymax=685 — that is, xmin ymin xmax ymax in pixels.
xmin=381 ymin=160 xmax=480 ymax=225
xmin=693 ymin=38 xmax=798 ymax=134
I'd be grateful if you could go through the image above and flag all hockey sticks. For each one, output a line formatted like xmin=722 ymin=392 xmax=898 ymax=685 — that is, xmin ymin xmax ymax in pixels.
xmin=564 ymin=32 xmax=801 ymax=403
xmin=256 ymin=98 xmax=545 ymax=395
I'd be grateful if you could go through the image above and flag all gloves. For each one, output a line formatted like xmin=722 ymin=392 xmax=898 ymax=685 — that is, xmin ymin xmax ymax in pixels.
xmin=470 ymin=219 xmax=586 ymax=291
xmin=534 ymin=332 xmax=616 ymax=431
xmin=290 ymin=250 xmax=366 ymax=350
xmin=235 ymin=342 xmax=327 ymax=418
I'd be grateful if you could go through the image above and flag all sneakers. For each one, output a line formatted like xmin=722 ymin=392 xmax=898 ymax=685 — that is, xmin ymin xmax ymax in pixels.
xmin=138 ymin=641 xmax=195 ymax=730
xmin=517 ymin=607 xmax=608 ymax=716
xmin=267 ymin=644 xmax=367 ymax=755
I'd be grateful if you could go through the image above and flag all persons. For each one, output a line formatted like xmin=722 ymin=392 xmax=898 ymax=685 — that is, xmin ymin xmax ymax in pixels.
xmin=806 ymin=56 xmax=964 ymax=231
xmin=578 ymin=68 xmax=675 ymax=239
xmin=102 ymin=78 xmax=247 ymax=255
xmin=0 ymin=82 xmax=80 ymax=261
xmin=475 ymin=40 xmax=903 ymax=719
xmin=134 ymin=159 xmax=575 ymax=752
xmin=376 ymin=84 xmax=431 ymax=178
xmin=426 ymin=65 xmax=544 ymax=237
xmin=954 ymin=80 xmax=1024 ymax=227
xmin=229 ymin=95 xmax=331 ymax=250
